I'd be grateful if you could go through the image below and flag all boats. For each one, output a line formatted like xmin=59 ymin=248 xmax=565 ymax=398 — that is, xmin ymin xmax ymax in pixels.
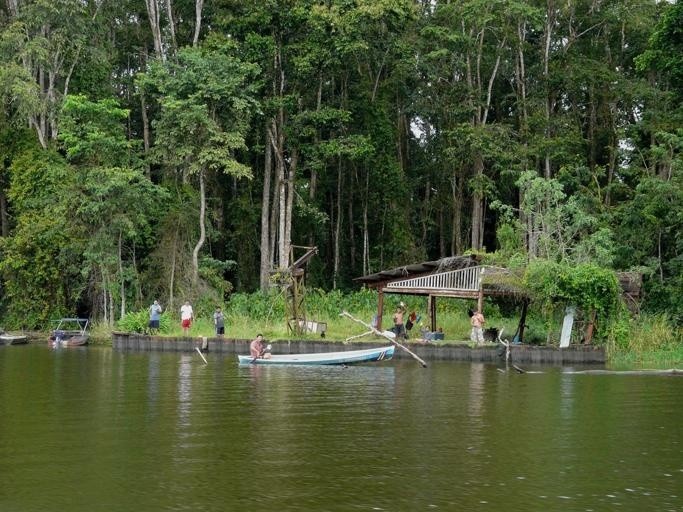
xmin=236 ymin=344 xmax=394 ymax=362
xmin=47 ymin=318 xmax=91 ymax=346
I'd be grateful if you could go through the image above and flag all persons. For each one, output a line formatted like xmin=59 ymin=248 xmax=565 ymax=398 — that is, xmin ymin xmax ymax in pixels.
xmin=214 ymin=306 xmax=226 ymax=335
xmin=471 ymin=309 xmax=486 ymax=341
xmin=249 ymin=334 xmax=272 ymax=359
xmin=393 ymin=301 xmax=409 ymax=339
xmin=180 ymin=300 xmax=195 ymax=336
xmin=144 ymin=299 xmax=163 ymax=336
xmin=419 ymin=322 xmax=435 ymax=340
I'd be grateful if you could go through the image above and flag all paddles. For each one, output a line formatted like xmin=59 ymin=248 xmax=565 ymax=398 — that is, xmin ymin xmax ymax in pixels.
xmin=250 ymin=344 xmax=273 ymax=364
